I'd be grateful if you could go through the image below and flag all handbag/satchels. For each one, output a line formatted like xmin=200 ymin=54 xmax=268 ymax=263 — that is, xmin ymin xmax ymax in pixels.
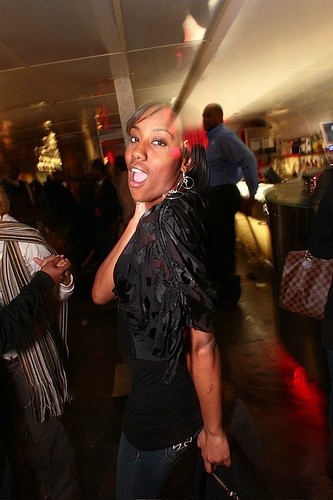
xmin=278 ymin=249 xmax=333 ymax=320
xmin=191 ymin=427 xmax=274 ymax=500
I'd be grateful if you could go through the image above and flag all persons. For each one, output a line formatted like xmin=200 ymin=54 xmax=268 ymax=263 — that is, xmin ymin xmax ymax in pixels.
xmin=93 ymin=94 xmax=232 ymax=500
xmin=0 ymin=105 xmax=333 ymax=500
